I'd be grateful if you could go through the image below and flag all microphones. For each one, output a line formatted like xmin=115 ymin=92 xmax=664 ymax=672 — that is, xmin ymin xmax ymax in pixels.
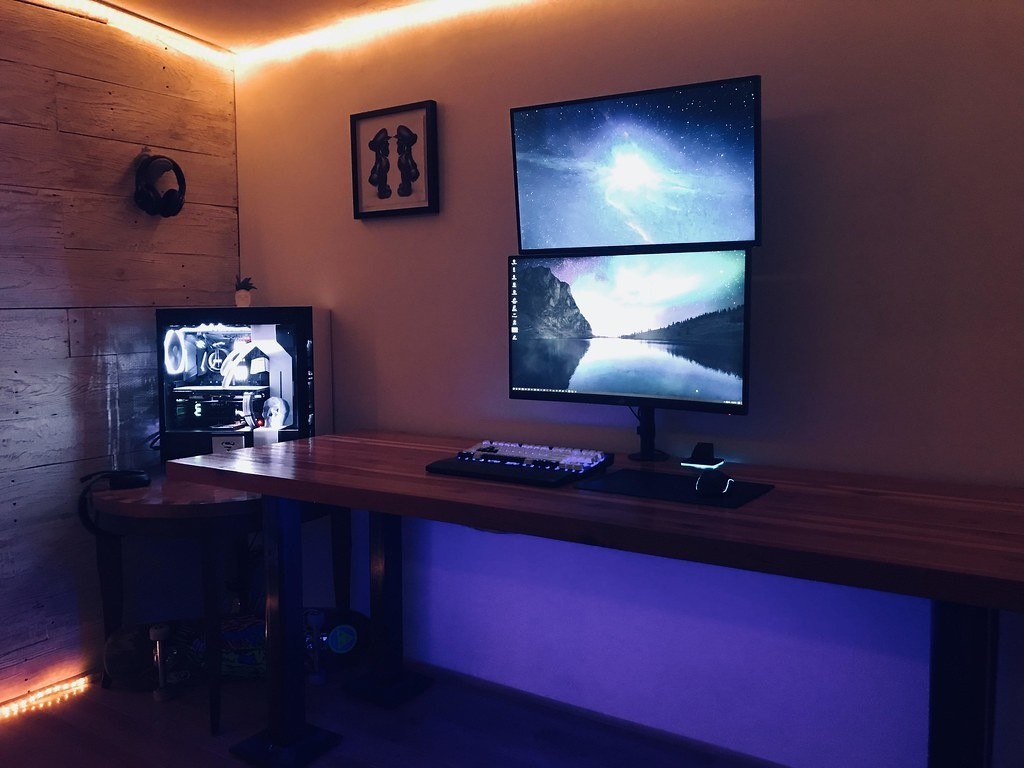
xmin=80 ymin=469 xmax=119 ymax=482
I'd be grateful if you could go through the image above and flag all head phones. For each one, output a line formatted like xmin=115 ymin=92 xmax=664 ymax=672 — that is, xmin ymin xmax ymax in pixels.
xmin=77 ymin=468 xmax=151 ymax=538
xmin=134 ymin=154 xmax=186 ymax=218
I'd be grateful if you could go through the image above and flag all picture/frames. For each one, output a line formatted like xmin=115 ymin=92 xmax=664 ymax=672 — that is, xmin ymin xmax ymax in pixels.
xmin=350 ymin=100 xmax=438 ymax=220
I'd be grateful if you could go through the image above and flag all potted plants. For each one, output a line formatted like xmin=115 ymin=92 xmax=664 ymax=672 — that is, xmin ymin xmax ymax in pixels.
xmin=234 ymin=274 xmax=258 ymax=306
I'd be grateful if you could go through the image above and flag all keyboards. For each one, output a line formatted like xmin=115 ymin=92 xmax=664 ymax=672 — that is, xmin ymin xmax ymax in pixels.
xmin=426 ymin=439 xmax=615 ymax=486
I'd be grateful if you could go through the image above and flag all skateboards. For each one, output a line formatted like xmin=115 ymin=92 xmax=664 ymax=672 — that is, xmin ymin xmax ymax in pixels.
xmin=103 ymin=605 xmax=375 ymax=706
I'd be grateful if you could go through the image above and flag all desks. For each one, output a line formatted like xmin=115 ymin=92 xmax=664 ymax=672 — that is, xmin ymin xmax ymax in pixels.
xmin=88 ymin=465 xmax=351 ymax=738
xmin=165 ymin=430 xmax=1024 ymax=768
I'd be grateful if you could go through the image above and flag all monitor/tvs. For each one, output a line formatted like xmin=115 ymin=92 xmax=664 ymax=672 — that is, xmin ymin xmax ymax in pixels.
xmin=510 ymin=76 xmax=762 ymax=256
xmin=507 ymin=245 xmax=751 ymax=464
xmin=154 ymin=306 xmax=314 ymax=455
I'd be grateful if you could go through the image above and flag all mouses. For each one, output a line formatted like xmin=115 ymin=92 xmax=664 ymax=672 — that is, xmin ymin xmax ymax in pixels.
xmin=694 ymin=469 xmax=735 ymax=497
